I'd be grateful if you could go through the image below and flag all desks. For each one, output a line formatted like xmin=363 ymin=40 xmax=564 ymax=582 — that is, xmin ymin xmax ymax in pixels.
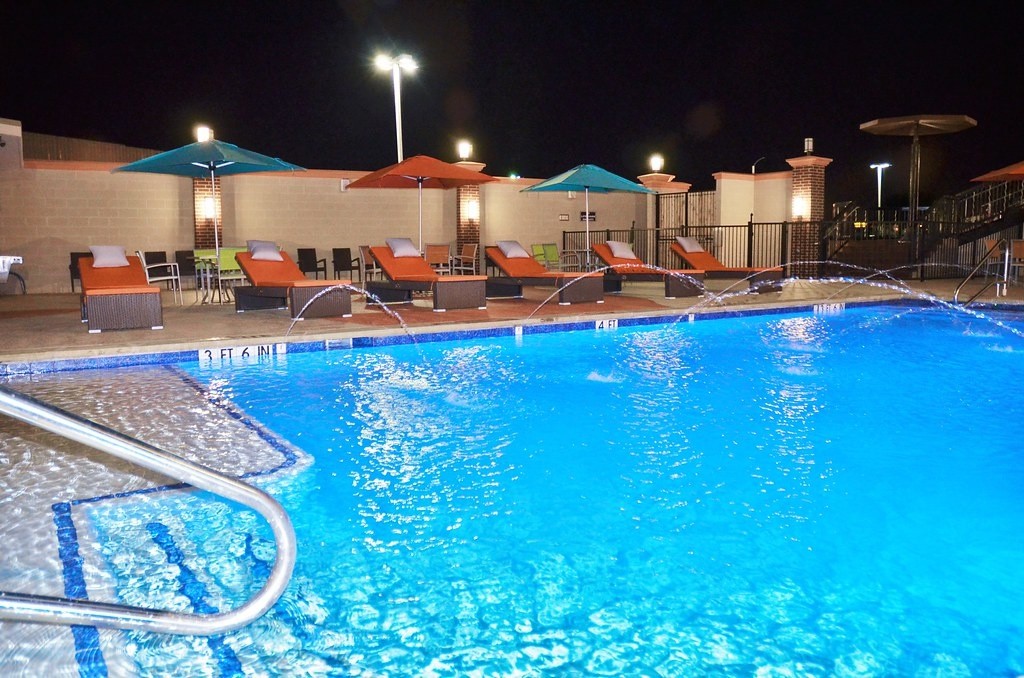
xmin=563 ymin=248 xmax=594 ymax=272
xmin=187 ymin=255 xmax=219 ymax=305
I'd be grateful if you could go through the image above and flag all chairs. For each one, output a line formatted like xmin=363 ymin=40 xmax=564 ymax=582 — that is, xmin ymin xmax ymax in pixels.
xmin=592 ymin=243 xmax=704 ymax=301
xmin=368 ymin=244 xmax=489 ymax=315
xmin=486 ymin=247 xmax=604 ymax=307
xmin=136 ymin=244 xmax=510 ymax=305
xmin=987 ymin=238 xmax=1024 ymax=285
xmin=671 ymin=242 xmax=783 ymax=297
xmin=531 ymin=243 xmax=609 ymax=272
xmin=77 ymin=255 xmax=165 ymax=334
xmin=69 ymin=250 xmax=95 ymax=292
xmin=234 ymin=252 xmax=354 ymax=323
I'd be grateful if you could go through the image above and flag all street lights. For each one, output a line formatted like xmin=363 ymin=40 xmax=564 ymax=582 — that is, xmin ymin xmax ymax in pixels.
xmin=373 ymin=53 xmax=416 ymax=164
xmin=870 ymin=162 xmax=890 ymax=221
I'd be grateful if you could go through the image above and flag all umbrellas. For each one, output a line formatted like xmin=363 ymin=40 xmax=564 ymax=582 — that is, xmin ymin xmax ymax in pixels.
xmin=971 ymin=161 xmax=1024 ymax=182
xmin=111 ymin=138 xmax=307 ymax=306
xmin=345 ymin=154 xmax=500 ymax=252
xmin=519 ymin=165 xmax=656 ymax=265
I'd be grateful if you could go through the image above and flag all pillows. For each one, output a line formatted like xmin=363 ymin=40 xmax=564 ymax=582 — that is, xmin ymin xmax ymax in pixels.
xmin=245 ymin=240 xmax=283 ymax=261
xmin=387 ymin=238 xmax=420 ymax=256
xmin=605 ymin=240 xmax=637 ymax=258
xmin=676 ymin=237 xmax=704 ymax=253
xmin=89 ymin=244 xmax=131 ymax=267
xmin=496 ymin=241 xmax=527 ymax=258
